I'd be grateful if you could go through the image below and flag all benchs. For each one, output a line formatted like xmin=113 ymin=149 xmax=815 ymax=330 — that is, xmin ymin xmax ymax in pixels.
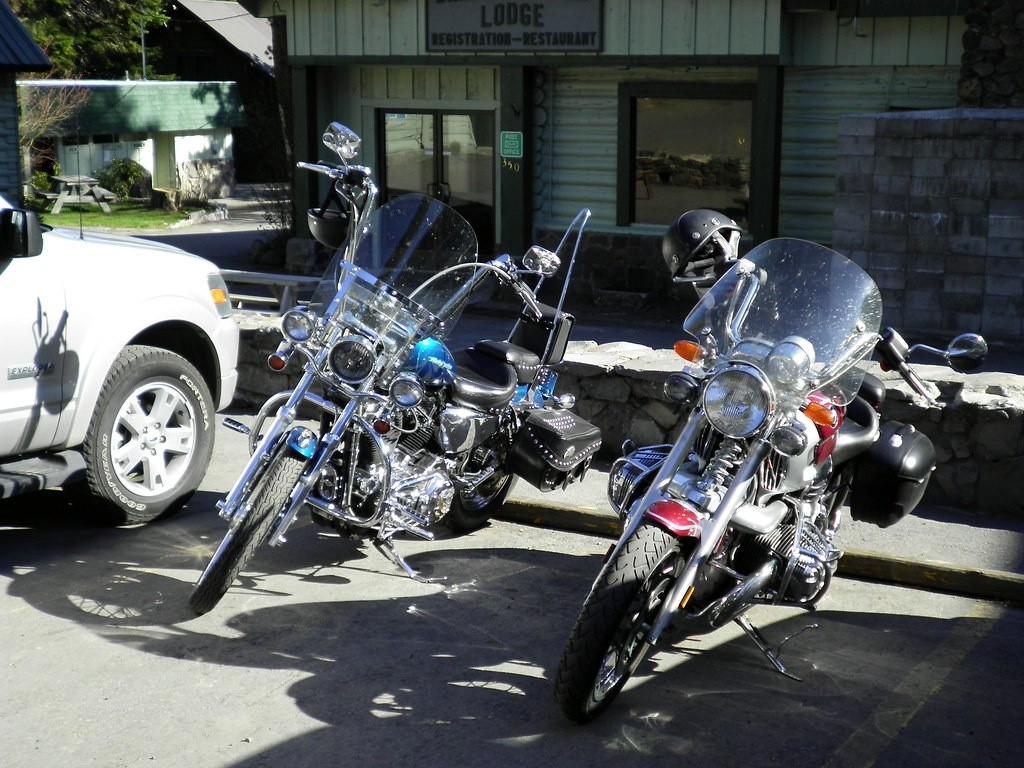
xmin=35 ymin=189 xmax=58 ymax=199
xmin=92 ymin=186 xmax=115 ymax=200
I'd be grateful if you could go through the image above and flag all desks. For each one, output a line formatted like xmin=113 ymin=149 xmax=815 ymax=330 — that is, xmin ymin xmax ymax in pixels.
xmin=50 ymin=176 xmax=110 ymax=214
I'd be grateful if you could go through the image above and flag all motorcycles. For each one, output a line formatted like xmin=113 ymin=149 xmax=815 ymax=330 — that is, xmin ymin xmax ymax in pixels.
xmin=188 ymin=120 xmax=603 ymax=617
xmin=552 ymin=236 xmax=990 ymax=724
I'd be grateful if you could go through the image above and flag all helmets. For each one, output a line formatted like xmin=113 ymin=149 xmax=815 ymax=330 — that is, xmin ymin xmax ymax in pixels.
xmin=307 ymin=208 xmax=351 ymax=250
xmin=662 ymin=208 xmax=743 ymax=279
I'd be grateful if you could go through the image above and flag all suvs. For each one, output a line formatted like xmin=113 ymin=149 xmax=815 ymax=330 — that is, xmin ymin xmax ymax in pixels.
xmin=0 ymin=195 xmax=240 ymax=529
